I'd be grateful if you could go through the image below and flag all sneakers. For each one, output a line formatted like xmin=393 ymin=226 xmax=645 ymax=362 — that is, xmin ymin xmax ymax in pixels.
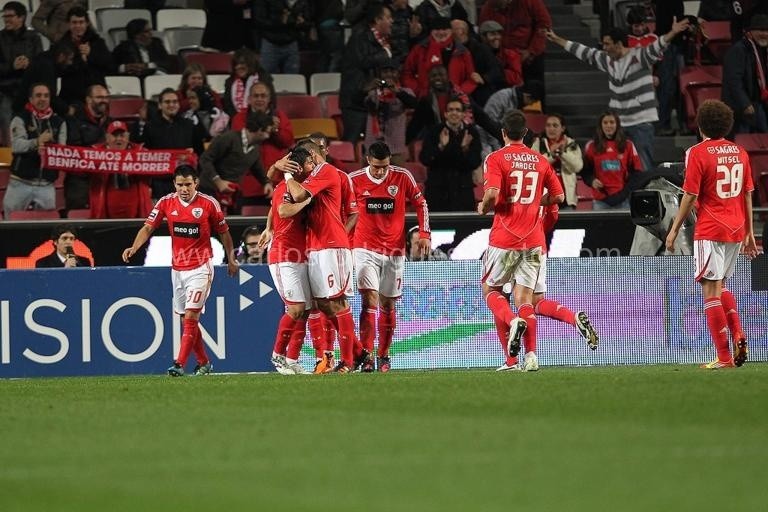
xmin=167 ymin=365 xmax=186 ymax=376
xmin=495 ymin=361 xmax=521 ymax=372
xmin=574 ymin=311 xmax=598 ymax=350
xmin=656 ymin=125 xmax=675 ymax=137
xmin=313 ymin=348 xmax=390 ymax=374
xmin=523 ymin=352 xmax=538 ymax=371
xmin=271 ymin=353 xmax=304 ymax=375
xmin=192 ymin=361 xmax=213 ymax=376
xmin=733 ymin=331 xmax=748 ymax=367
xmin=700 ymin=356 xmax=735 ymax=369
xmin=507 ymin=318 xmax=527 ymax=357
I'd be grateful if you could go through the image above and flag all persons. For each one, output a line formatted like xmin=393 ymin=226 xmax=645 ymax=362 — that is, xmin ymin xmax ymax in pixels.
xmin=661 ymin=99 xmax=761 ymax=368
xmin=535 ymin=110 xmax=643 ymax=211
xmin=256 ymin=129 xmax=379 ymax=375
xmin=477 ymin=109 xmax=569 ymax=374
xmin=405 ymin=226 xmax=447 ymax=261
xmin=543 ymin=15 xmax=689 ymax=168
xmin=344 ymin=141 xmax=434 ymax=374
xmin=1 ymin=1 xmax=299 ymax=214
xmin=623 ymin=2 xmax=768 ymax=133
xmin=123 ymin=164 xmax=243 ymax=379
xmin=36 ymin=223 xmax=92 ymax=267
xmin=290 ymin=1 xmax=553 ymax=211
xmin=492 ymin=199 xmax=599 ymax=371
xmin=237 ymin=226 xmax=268 ymax=263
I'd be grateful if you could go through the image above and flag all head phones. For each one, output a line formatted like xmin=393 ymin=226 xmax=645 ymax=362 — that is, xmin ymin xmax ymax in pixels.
xmin=236 ymin=226 xmax=258 ymax=255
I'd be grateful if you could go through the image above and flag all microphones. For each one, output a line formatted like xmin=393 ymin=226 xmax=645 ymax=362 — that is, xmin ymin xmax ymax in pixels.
xmin=67 ymin=246 xmax=75 ymax=258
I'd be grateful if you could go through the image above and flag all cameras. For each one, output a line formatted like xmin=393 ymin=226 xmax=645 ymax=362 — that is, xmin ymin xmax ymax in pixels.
xmin=381 ymin=80 xmax=388 ymax=87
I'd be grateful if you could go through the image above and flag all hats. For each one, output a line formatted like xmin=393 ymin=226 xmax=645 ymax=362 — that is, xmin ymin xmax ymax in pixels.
xmin=430 ymin=17 xmax=451 ymax=29
xmin=108 ymin=121 xmax=129 ymax=133
xmin=383 ymin=58 xmax=399 ymax=69
xmin=478 ymin=20 xmax=503 ymax=33
xmin=748 ymin=14 xmax=768 ymax=30
